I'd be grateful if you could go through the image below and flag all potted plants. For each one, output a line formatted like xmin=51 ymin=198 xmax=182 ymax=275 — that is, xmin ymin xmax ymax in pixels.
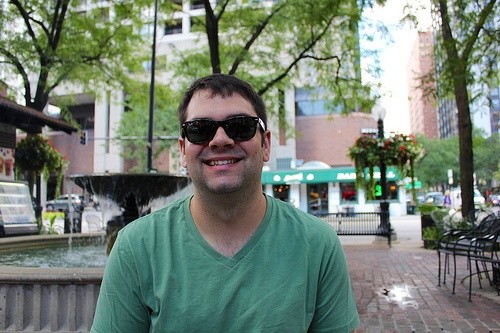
xmin=417 ymin=204 xmax=447 ymax=250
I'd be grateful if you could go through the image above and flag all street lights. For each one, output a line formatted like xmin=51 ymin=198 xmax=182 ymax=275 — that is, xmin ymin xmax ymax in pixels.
xmin=371 ymin=104 xmax=394 ymax=234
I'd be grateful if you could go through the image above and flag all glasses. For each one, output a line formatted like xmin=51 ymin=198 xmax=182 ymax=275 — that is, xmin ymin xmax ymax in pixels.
xmin=181 ymin=116 xmax=266 ymax=144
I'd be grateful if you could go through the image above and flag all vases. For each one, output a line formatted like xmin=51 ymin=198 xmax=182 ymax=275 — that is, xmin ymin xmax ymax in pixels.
xmin=390 ymin=157 xmax=407 ymax=166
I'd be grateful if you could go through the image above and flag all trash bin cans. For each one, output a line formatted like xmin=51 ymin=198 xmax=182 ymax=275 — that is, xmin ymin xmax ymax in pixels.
xmin=63 ymin=205 xmax=84 ymax=233
xmin=418 ymin=204 xmax=452 ymax=251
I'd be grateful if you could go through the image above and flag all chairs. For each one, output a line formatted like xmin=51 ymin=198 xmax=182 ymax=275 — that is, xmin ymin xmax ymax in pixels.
xmin=437 ymin=207 xmax=500 ymax=303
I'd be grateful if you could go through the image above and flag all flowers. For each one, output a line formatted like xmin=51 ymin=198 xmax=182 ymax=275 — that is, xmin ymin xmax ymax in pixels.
xmin=13 ymin=133 xmax=70 ymax=198
xmin=348 ymin=134 xmax=423 ymax=207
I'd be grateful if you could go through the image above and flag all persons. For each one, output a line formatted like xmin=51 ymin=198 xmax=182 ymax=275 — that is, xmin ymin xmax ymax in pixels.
xmin=443 ymin=190 xmax=452 ymax=208
xmin=89 ymin=73 xmax=360 ymax=333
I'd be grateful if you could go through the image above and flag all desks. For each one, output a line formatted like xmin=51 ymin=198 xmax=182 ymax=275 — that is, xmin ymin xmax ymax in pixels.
xmin=311 ymin=211 xmax=395 ymax=247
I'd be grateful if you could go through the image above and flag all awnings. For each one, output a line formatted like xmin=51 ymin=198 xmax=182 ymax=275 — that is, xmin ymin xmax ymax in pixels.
xmin=261 ymin=164 xmax=399 ymax=185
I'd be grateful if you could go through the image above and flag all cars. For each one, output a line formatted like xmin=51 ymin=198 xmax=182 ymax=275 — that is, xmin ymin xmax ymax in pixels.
xmin=46 ymin=194 xmax=84 ymax=212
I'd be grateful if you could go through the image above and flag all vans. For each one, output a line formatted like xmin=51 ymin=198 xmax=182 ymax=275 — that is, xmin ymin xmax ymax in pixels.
xmin=423 ymin=187 xmax=500 ymax=211
